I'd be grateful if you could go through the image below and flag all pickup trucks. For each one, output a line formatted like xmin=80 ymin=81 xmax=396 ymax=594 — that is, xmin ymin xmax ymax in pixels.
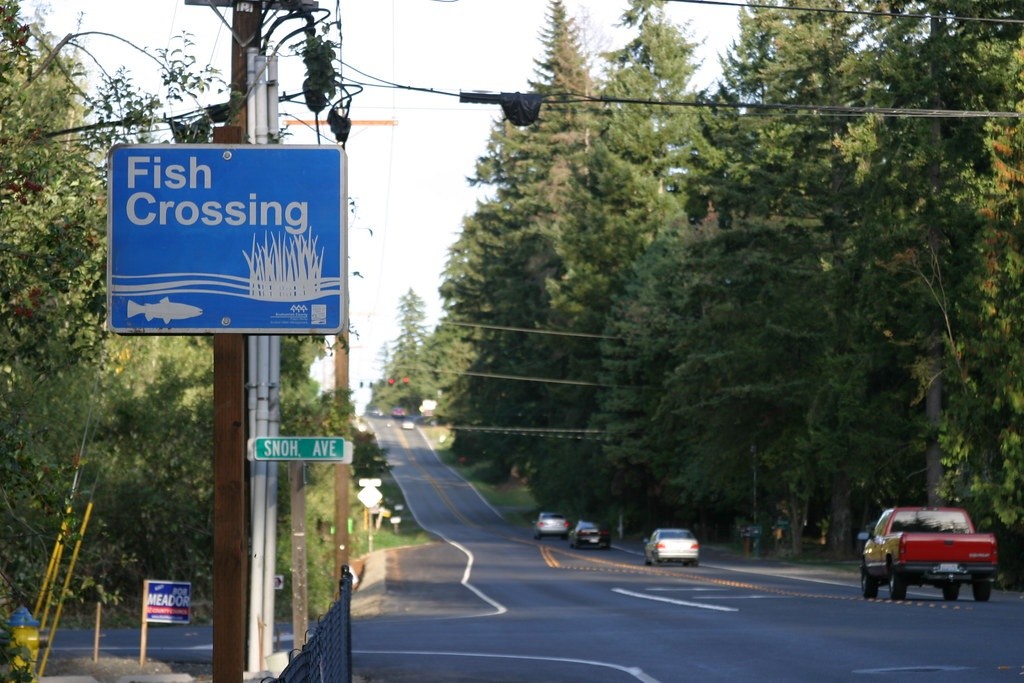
xmin=856 ymin=505 xmax=997 ymax=601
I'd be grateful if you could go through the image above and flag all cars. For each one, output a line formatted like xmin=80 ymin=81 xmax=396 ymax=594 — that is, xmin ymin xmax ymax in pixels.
xmin=644 ymin=528 xmax=699 ymax=566
xmin=369 ymin=409 xmax=384 ymax=417
xmin=569 ymin=520 xmax=610 ymax=550
xmin=392 ymin=407 xmax=406 ymax=417
xmin=402 ymin=420 xmax=414 ymax=429
xmin=532 ymin=513 xmax=570 ymax=539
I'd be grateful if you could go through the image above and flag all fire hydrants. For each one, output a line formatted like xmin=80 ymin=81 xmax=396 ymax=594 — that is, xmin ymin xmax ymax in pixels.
xmin=7 ymin=603 xmax=49 ymax=683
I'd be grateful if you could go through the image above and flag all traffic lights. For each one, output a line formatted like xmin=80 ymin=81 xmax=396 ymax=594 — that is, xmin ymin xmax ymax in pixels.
xmin=403 ymin=376 xmax=408 ymax=385
xmin=388 ymin=377 xmax=394 ymax=385
